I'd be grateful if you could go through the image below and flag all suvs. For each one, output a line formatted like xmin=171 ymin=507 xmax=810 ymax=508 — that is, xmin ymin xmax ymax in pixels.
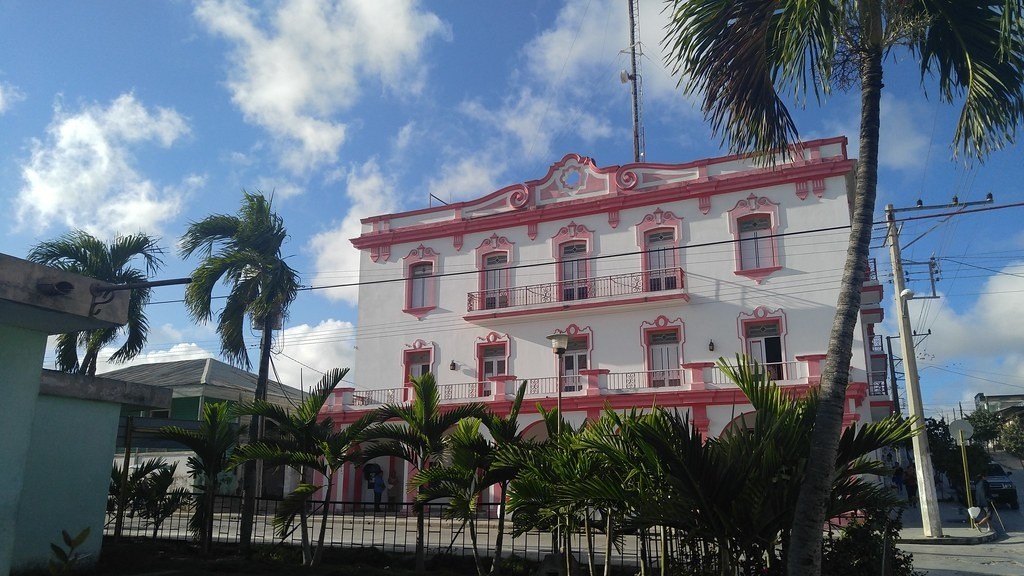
xmin=956 ymin=460 xmax=1019 ymax=511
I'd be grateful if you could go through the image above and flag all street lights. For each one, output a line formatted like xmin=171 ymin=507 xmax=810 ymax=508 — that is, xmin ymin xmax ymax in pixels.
xmin=546 ymin=331 xmax=573 ymax=458
xmin=882 ymin=204 xmax=943 ymax=538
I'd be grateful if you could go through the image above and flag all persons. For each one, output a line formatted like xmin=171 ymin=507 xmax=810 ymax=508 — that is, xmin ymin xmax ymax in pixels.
xmin=974 ymin=472 xmax=996 ymax=534
xmin=386 ymin=469 xmax=400 ymax=513
xmin=372 ymin=470 xmax=386 ymax=512
xmin=892 ymin=460 xmax=918 ymax=510
